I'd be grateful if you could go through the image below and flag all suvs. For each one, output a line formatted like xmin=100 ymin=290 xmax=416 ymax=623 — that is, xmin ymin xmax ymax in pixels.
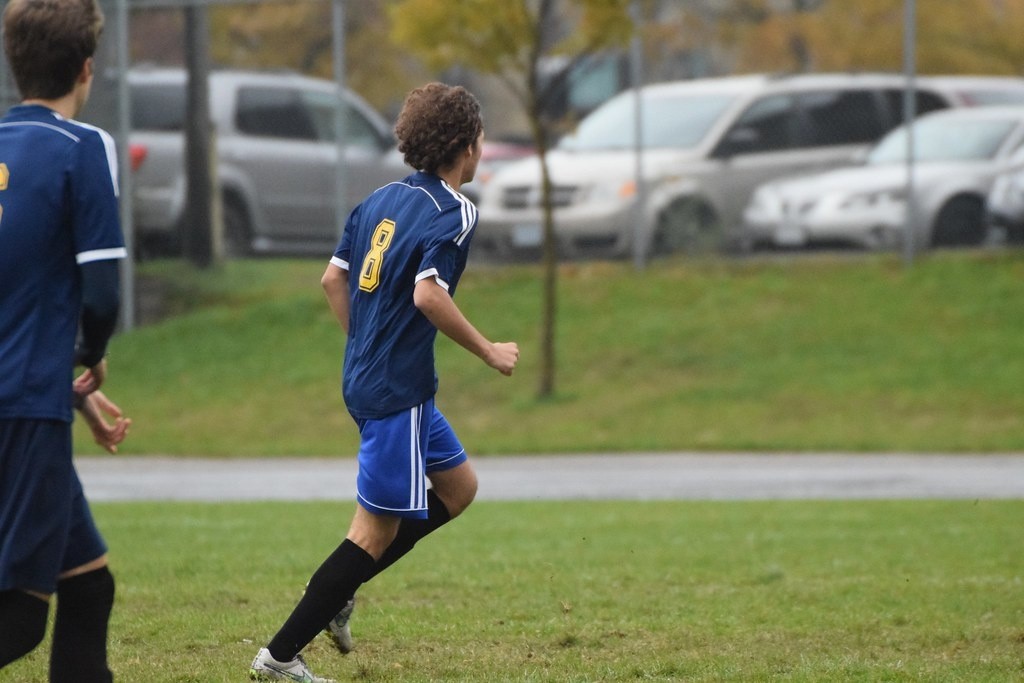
xmin=80 ymin=59 xmax=418 ymax=269
xmin=461 ymin=67 xmax=980 ymax=273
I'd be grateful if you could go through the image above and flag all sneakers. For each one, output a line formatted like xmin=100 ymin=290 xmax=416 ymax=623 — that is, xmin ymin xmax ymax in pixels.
xmin=249 ymin=648 xmax=337 ymax=683
xmin=304 ymin=582 xmax=358 ymax=651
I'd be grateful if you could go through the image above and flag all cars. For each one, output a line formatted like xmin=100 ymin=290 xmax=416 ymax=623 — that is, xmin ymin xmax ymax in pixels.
xmin=741 ymin=105 xmax=1024 ymax=259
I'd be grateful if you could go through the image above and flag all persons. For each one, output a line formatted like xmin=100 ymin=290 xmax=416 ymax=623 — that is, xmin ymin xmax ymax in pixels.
xmin=0 ymin=0 xmax=131 ymax=683
xmin=248 ymin=83 xmax=520 ymax=682
